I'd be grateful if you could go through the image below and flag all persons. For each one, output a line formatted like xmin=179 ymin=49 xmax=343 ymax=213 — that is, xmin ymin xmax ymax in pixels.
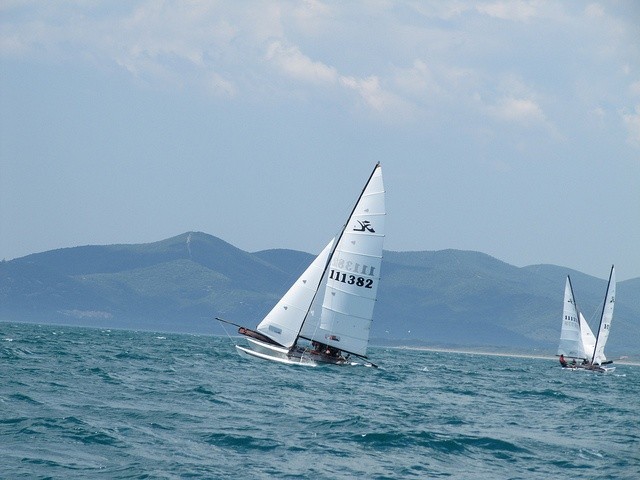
xmin=582 ymin=359 xmax=588 ymax=365
xmin=570 ymin=358 xmax=577 ymax=366
xmin=312 ymin=333 xmax=339 ymax=356
xmin=559 ymin=354 xmax=567 ymax=367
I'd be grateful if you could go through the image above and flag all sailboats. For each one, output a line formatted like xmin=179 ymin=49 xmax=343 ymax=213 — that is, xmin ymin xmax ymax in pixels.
xmin=556 ymin=263 xmax=620 ymax=374
xmin=214 ymin=160 xmax=386 ymax=369
